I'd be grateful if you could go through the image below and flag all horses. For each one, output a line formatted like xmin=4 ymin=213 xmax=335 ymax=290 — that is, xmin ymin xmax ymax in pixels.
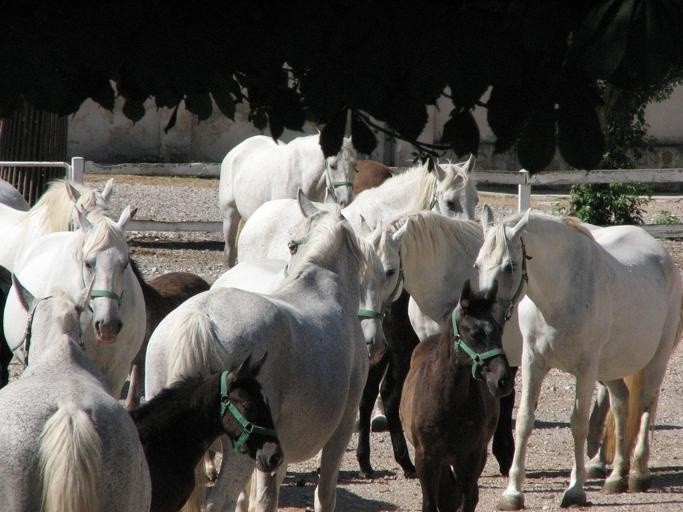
xmin=0 ymin=127 xmax=683 ymax=512
